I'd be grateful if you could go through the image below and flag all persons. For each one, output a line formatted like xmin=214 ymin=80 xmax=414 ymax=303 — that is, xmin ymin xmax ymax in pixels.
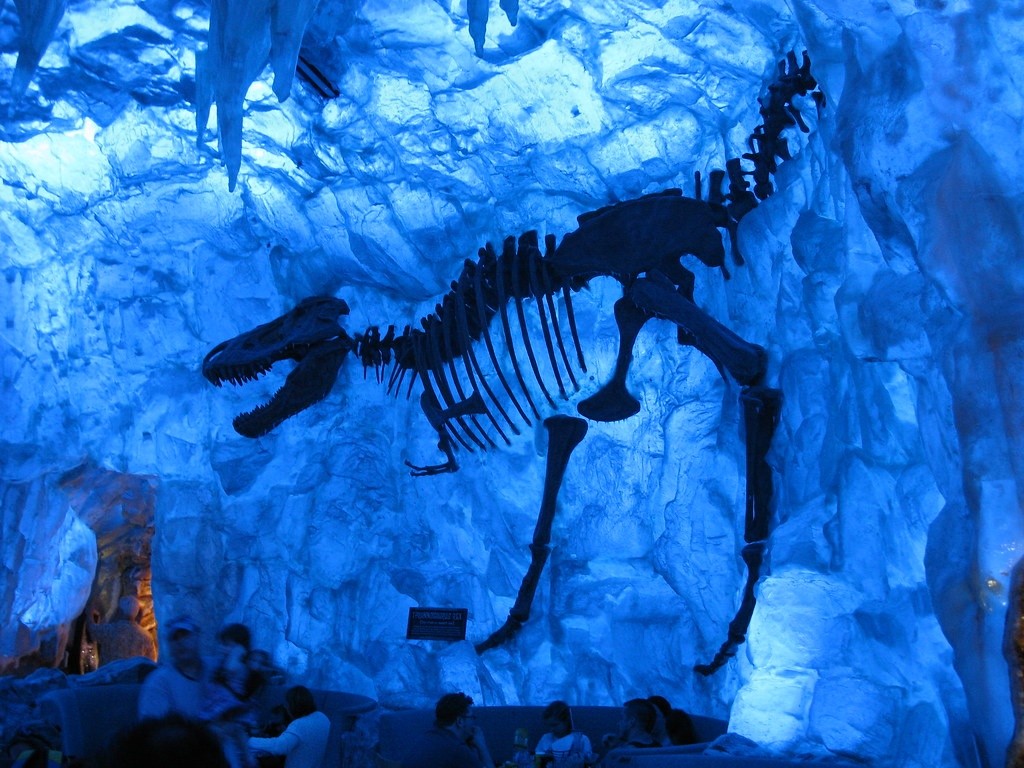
xmin=85 ymin=596 xmax=156 ymax=669
xmin=398 ymin=692 xmax=495 ymax=768
xmin=198 ymin=623 xmax=265 ymax=767
xmin=534 ymin=701 xmax=593 ymax=768
xmin=105 ymin=709 xmax=231 ymax=768
xmin=249 ymin=686 xmax=330 ymax=768
xmin=138 ymin=614 xmax=219 ymax=723
xmin=596 ymin=696 xmax=700 ymax=768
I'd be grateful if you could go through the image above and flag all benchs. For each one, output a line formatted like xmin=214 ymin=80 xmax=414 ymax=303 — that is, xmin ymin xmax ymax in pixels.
xmin=375 ymin=703 xmax=728 ymax=768
xmin=54 ymin=682 xmax=375 ymax=768
